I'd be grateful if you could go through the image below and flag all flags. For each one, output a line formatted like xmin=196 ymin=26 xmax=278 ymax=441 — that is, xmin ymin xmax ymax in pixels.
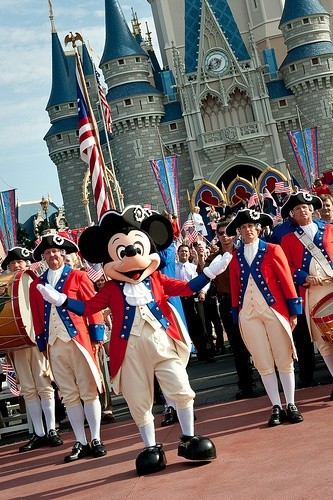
xmin=275 ymin=181 xmax=290 ymax=193
xmin=93 ymin=66 xmax=112 ymax=134
xmin=249 ymin=188 xmax=277 ymax=208
xmin=0 ymin=355 xmax=22 ymax=396
xmin=74 ymin=64 xmax=110 ymax=224
xmin=180 ymin=219 xmax=202 ymax=244
xmin=87 ymin=264 xmax=103 ymax=283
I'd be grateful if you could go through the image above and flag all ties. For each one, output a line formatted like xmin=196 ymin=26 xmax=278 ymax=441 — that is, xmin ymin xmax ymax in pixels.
xmin=179 ymin=262 xmax=188 ymax=280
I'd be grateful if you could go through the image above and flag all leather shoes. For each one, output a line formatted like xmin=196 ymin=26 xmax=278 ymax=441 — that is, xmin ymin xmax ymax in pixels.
xmin=91 ymin=439 xmax=105 ymax=457
xmin=47 ymin=429 xmax=63 ymax=446
xmin=161 ymin=410 xmax=178 ymax=426
xmin=19 ymin=432 xmax=47 ymax=451
xmin=268 ymin=404 xmax=287 ymax=425
xmin=65 ymin=441 xmax=91 ymax=461
xmin=286 ymin=403 xmax=303 ymax=422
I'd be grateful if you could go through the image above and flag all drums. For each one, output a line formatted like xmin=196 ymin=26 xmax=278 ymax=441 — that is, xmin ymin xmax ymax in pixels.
xmin=0 ymin=270 xmax=39 ymax=350
xmin=310 ymin=292 xmax=333 ymax=348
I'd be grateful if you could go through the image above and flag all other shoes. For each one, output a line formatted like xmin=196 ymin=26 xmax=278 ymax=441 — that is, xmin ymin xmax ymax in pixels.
xmin=234 ymin=389 xmax=253 ymax=399
xmin=103 ymin=413 xmax=115 ymax=424
xmin=215 ymin=345 xmax=225 ymax=354
xmin=298 ymin=378 xmax=313 ymax=387
xmin=197 ymin=352 xmax=205 ymax=361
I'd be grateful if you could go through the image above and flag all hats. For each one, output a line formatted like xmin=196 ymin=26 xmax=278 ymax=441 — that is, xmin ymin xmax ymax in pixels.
xmin=31 ymin=235 xmax=80 ymax=262
xmin=1 ymin=247 xmax=35 ymax=270
xmin=281 ymin=190 xmax=323 ymax=220
xmin=226 ymin=209 xmax=275 ymax=237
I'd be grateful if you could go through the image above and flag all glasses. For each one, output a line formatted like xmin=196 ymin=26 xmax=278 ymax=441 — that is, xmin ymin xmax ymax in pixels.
xmin=217 ymin=231 xmax=225 ymax=236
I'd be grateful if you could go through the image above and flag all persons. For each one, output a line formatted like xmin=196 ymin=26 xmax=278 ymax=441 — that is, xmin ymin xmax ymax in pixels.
xmin=167 ymin=213 xmax=181 ymax=241
xmin=1 ymin=247 xmax=62 ymax=453
xmin=206 ymin=205 xmax=221 ymax=239
xmin=293 ymin=185 xmax=299 ymax=191
xmin=175 ymin=241 xmax=226 ymax=364
xmin=225 ymin=208 xmax=305 ymax=427
xmin=157 ymin=241 xmax=197 ymax=426
xmin=28 ymin=235 xmax=117 ymax=464
xmin=192 ymin=205 xmax=208 ymax=241
xmin=311 ymin=176 xmax=332 ymax=197
xmin=193 ymin=220 xmax=266 ymax=399
xmin=280 ymin=190 xmax=333 ymax=401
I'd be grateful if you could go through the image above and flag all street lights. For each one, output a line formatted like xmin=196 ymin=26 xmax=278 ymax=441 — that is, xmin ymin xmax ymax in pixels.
xmin=39 ymin=195 xmax=52 ymax=234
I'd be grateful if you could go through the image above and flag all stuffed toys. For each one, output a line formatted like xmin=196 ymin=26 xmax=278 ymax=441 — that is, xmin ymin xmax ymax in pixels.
xmin=37 ymin=204 xmax=233 ymax=475
